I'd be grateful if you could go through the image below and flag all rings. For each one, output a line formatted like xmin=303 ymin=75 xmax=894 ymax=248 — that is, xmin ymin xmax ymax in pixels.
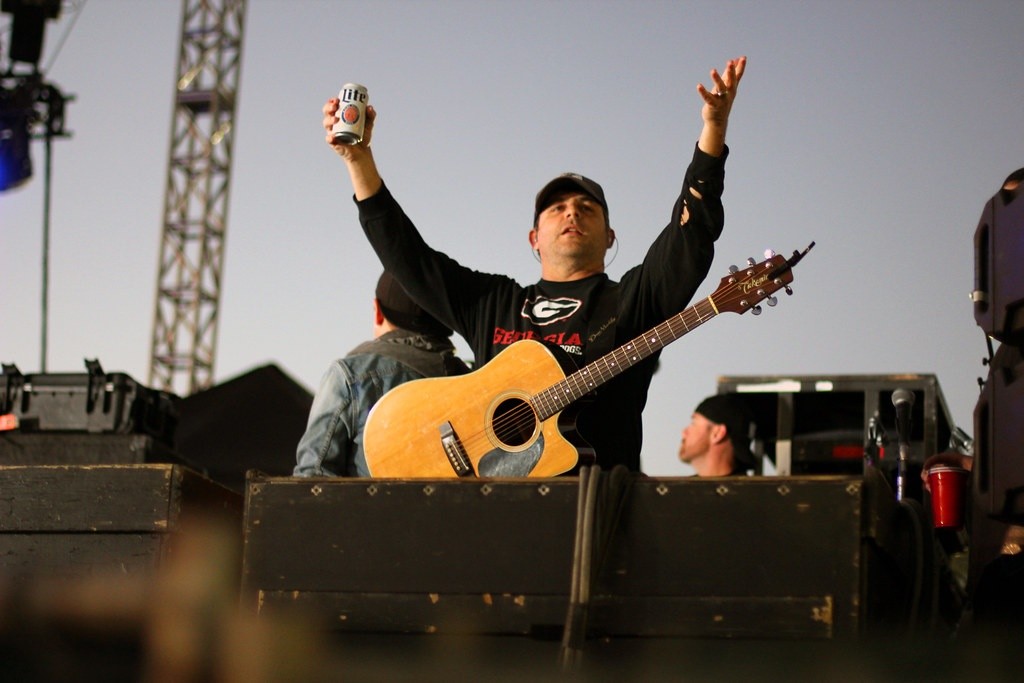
xmin=715 ymin=86 xmax=729 ymax=97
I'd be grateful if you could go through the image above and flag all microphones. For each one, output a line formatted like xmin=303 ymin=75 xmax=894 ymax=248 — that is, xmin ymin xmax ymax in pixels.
xmin=890 ymin=387 xmax=916 ymax=468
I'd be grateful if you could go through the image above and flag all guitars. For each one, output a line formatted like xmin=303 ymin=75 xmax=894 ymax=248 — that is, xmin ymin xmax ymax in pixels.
xmin=362 ymin=240 xmax=815 ymax=478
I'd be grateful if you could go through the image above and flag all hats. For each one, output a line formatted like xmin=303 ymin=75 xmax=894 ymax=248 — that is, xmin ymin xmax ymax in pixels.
xmin=696 ymin=390 xmax=753 ymax=447
xmin=375 ymin=268 xmax=454 ymax=338
xmin=534 ymin=171 xmax=608 ymax=217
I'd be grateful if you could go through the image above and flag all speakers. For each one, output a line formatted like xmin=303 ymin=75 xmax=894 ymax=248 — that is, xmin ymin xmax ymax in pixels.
xmin=971 ymin=166 xmax=1024 ymax=527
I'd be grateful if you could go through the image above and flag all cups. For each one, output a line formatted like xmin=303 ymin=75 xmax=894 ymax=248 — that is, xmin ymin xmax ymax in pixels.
xmin=929 ymin=465 xmax=969 ymax=529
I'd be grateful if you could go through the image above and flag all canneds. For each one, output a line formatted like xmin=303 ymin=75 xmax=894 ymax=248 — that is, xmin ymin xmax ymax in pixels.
xmin=333 ymin=85 xmax=369 ymax=145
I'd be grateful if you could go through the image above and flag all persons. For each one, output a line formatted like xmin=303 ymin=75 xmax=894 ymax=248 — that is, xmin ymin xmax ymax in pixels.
xmin=323 ymin=55 xmax=748 ymax=477
xmin=679 ymin=392 xmax=782 ymax=478
xmin=293 ymin=269 xmax=477 ymax=478
